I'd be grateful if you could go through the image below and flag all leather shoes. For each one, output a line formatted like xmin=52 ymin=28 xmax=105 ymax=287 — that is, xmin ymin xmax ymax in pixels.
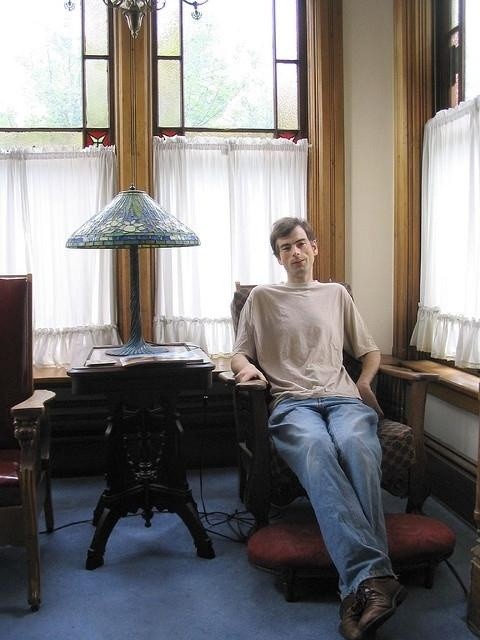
xmin=338 ymin=576 xmax=408 ymax=640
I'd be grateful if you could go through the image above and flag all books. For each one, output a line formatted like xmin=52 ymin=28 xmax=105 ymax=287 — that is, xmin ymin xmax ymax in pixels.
xmin=121 ymin=351 xmax=206 ymax=368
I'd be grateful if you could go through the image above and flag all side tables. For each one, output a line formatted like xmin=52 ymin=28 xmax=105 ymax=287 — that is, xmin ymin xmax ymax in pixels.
xmin=67 ymin=342 xmax=216 ymax=570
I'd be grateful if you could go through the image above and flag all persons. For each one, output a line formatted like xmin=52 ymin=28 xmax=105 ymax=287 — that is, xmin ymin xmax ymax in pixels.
xmin=229 ymin=218 xmax=411 ymax=639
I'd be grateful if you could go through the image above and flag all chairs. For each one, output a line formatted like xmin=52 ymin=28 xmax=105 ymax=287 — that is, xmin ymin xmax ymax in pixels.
xmin=0 ymin=273 xmax=56 ymax=613
xmin=218 ymin=282 xmax=440 ymax=537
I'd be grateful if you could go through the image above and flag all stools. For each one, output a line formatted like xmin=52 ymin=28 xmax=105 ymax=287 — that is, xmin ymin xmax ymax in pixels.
xmin=246 ymin=513 xmax=455 ymax=603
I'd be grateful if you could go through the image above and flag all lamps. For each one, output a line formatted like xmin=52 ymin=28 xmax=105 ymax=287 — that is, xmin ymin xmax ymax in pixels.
xmin=64 ymin=0 xmax=206 ymax=40
xmin=65 ymin=185 xmax=200 ymax=357
